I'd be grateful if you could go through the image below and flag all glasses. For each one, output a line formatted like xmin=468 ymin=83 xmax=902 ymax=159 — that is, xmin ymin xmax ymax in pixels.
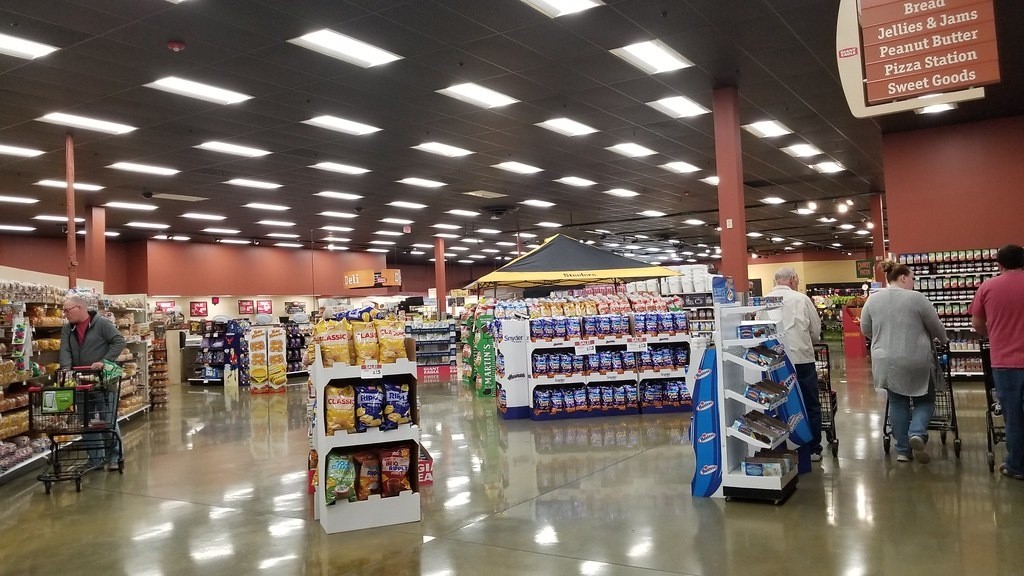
xmin=791 ymin=276 xmax=801 ymax=284
xmin=63 ymin=305 xmax=81 ymax=314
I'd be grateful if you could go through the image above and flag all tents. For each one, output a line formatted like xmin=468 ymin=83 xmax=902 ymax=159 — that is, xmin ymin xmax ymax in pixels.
xmin=461 ymin=234 xmax=684 ymax=300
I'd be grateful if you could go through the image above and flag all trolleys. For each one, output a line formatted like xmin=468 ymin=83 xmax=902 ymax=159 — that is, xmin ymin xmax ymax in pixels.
xmin=864 ymin=339 xmax=963 ymax=457
xmin=978 ymin=337 xmax=1007 ymax=473
xmin=810 ymin=343 xmax=840 ymax=458
xmin=26 ymin=365 xmax=125 ymax=495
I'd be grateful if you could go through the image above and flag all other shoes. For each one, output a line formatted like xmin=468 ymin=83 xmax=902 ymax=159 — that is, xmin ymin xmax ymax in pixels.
xmin=108 ymin=462 xmax=119 ymax=469
xmin=92 ymin=458 xmax=104 ymax=469
xmin=909 ymin=435 xmax=931 ymax=464
xmin=999 ymin=464 xmax=1024 ymax=480
xmin=811 ymin=448 xmax=823 ymax=462
xmin=897 ymin=448 xmax=912 ymax=461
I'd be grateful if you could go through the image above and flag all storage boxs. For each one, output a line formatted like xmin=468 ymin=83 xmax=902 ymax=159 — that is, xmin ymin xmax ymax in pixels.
xmin=713 ymin=276 xmax=796 ymax=477
xmin=417 ymin=442 xmax=434 ymax=486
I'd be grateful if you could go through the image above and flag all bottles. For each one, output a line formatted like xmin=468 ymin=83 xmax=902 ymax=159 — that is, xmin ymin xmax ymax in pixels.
xmin=950 ymin=355 xmax=983 ymax=372
xmin=706 ymin=296 xmax=712 ymax=306
xmin=495 ymin=300 xmax=527 ymax=319
xmin=688 ymin=310 xmax=713 ymax=320
xmin=495 ymin=353 xmax=505 ymax=378
xmin=486 ymin=296 xmax=497 ymax=315
xmin=496 ymin=384 xmax=507 ymax=413
xmin=287 ymin=326 xmax=300 ymax=372
xmin=492 ymin=318 xmax=504 ymax=342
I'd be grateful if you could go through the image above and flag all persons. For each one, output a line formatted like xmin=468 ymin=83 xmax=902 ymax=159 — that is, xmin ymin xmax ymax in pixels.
xmin=755 ymin=268 xmax=824 ymax=462
xmin=859 ymin=260 xmax=945 ymax=462
xmin=748 ymin=281 xmax=754 ymax=297
xmin=967 ymin=243 xmax=1024 ymax=481
xmin=59 ymin=295 xmax=125 ymax=470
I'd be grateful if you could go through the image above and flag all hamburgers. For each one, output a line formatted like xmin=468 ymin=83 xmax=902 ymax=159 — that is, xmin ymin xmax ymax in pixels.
xmin=251 ymin=368 xmax=268 ymax=384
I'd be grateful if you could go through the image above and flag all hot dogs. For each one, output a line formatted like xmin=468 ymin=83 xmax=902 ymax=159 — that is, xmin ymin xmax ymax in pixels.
xmin=268 ymin=367 xmax=286 ymax=385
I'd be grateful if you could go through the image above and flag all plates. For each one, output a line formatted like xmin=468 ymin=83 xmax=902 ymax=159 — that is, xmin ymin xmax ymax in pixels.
xmin=292 ymin=313 xmax=307 ymax=322
xmin=255 ymin=314 xmax=273 ymax=323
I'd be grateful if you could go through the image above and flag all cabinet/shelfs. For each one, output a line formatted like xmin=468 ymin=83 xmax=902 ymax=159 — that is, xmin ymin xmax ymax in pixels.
xmin=286 ymin=327 xmax=308 ymax=374
xmin=312 ymin=323 xmax=422 ymax=535
xmin=493 ymin=310 xmax=701 ymax=420
xmin=411 ymin=321 xmax=460 ymax=377
xmin=0 ymin=293 xmax=167 ymax=476
xmin=189 ymin=320 xmax=231 ymax=385
xmin=712 ymin=303 xmax=796 ymax=492
xmin=887 ymin=251 xmax=1006 ymax=376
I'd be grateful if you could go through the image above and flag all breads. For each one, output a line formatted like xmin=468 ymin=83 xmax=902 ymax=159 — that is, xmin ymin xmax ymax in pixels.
xmin=250 ymin=331 xmax=284 ymax=365
xmin=0 ymin=281 xmax=142 ymax=441
